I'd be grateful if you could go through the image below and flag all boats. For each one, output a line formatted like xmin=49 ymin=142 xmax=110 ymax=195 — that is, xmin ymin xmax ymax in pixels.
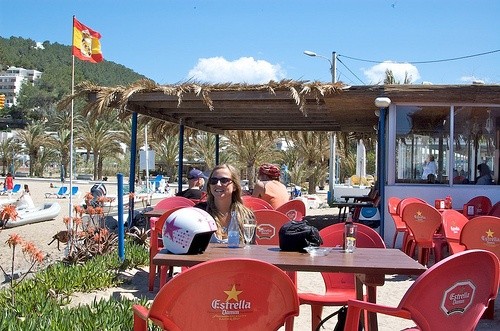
xmin=0 ymin=191 xmax=61 ymax=228
xmin=72 ymin=177 xmax=176 ymax=230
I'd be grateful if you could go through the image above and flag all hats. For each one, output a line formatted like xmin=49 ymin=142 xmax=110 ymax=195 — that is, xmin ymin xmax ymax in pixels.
xmin=259 ymin=162 xmax=280 ymax=179
xmin=187 ymin=168 xmax=209 ymax=179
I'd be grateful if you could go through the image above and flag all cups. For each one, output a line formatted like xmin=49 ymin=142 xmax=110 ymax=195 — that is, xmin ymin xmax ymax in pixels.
xmin=344 ymin=224 xmax=358 ymax=253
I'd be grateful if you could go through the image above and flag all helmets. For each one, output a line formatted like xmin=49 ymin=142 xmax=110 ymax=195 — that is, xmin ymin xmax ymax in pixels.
xmin=162 ymin=207 xmax=217 ymax=256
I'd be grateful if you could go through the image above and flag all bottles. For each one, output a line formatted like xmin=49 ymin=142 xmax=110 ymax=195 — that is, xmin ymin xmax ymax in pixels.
xmin=227 ymin=211 xmax=240 ymax=248
xmin=342 ymin=213 xmax=354 ymax=252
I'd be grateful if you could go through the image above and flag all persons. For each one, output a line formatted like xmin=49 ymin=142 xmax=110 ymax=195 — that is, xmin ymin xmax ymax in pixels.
xmin=12 ymin=184 xmax=35 ymax=211
xmin=421 ymin=154 xmax=437 ymax=184
xmin=192 ymin=164 xmax=258 ymax=245
xmin=251 ymin=162 xmax=290 ymax=211
xmin=172 ymin=168 xmax=207 ymax=201
xmin=474 ymin=164 xmax=493 ymax=183
xmin=0 ymin=173 xmax=14 ymax=190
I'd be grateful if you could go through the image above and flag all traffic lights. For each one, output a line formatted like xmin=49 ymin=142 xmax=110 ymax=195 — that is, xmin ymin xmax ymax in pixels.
xmin=0 ymin=95 xmax=5 ymax=110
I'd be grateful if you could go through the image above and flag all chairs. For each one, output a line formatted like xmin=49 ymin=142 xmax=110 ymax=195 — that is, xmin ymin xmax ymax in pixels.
xmin=57 ymin=187 xmax=78 ymax=199
xmin=45 ymin=187 xmax=67 ymax=198
xmin=0 ymin=184 xmax=22 ymax=195
xmin=130 ymin=175 xmax=500 ymax=331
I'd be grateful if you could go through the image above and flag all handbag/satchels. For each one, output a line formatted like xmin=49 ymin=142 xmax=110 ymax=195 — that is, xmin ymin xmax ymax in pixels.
xmin=316 ymin=304 xmax=363 ymax=331
xmin=279 ymin=219 xmax=322 ymax=252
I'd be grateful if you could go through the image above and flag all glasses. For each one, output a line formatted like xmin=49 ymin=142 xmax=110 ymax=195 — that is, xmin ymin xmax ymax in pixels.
xmin=208 ymin=176 xmax=233 ymax=185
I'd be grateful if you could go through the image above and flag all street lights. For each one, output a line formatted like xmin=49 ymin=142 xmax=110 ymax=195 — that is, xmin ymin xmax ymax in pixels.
xmin=303 ymin=50 xmax=337 ymax=209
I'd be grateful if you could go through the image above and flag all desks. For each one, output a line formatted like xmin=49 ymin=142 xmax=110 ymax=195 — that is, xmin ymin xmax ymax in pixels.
xmin=434 ymin=207 xmax=483 ymax=263
xmin=146 ymin=208 xmax=173 ymax=278
xmin=152 ymin=241 xmax=428 ymax=331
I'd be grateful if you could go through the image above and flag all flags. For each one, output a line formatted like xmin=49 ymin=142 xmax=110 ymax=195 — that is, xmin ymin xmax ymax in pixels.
xmin=72 ymin=17 xmax=103 ymax=64
